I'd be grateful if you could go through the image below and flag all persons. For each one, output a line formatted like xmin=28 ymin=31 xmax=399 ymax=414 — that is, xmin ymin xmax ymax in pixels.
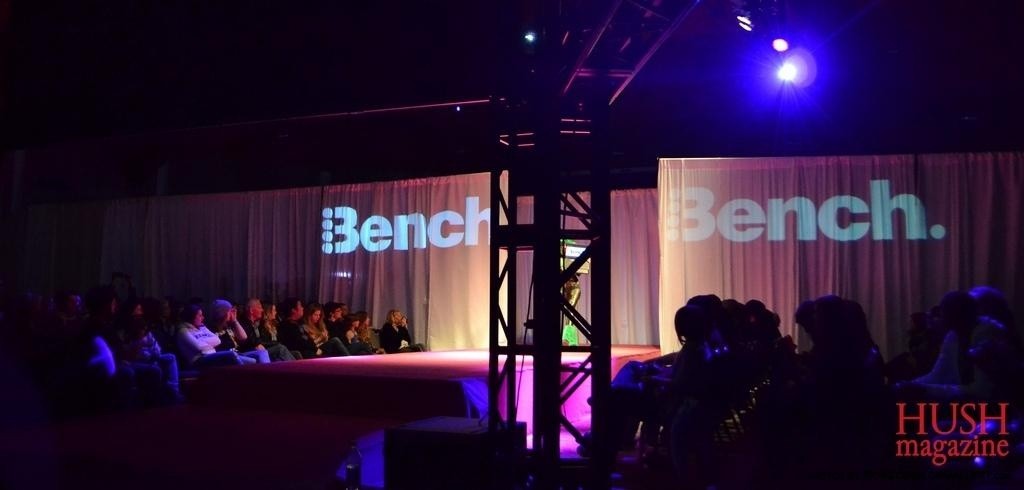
xmin=563 ymin=275 xmax=581 ymax=349
xmin=579 ymin=286 xmax=1024 ymax=489
xmin=49 ymin=278 xmax=427 ymax=407
